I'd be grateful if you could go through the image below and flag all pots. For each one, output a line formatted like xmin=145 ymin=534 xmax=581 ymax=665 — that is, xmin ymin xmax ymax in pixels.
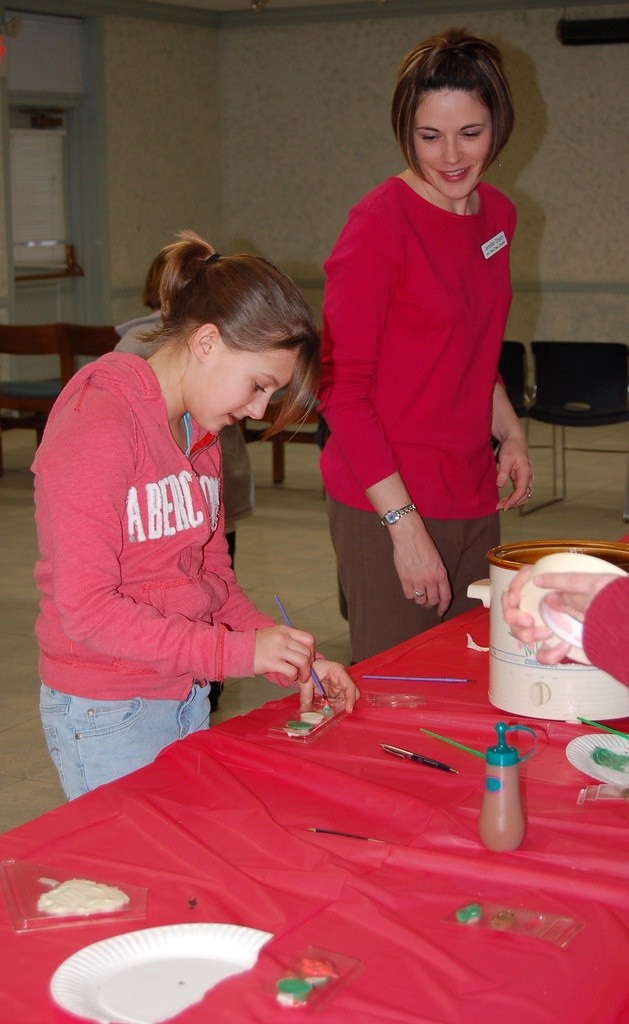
xmin=467 ymin=540 xmax=629 ymax=724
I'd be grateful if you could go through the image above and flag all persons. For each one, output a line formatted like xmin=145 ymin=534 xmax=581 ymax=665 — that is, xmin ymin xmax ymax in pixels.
xmin=501 ymin=564 xmax=629 ymax=689
xmin=29 ymin=235 xmax=359 ymax=802
xmin=314 ymin=30 xmax=534 ymax=664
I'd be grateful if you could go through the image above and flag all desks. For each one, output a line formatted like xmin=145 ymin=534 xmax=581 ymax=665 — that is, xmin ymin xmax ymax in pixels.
xmin=0 ymin=604 xmax=629 ymax=1024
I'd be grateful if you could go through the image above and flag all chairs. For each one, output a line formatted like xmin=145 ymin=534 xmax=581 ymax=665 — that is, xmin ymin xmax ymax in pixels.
xmin=0 ymin=322 xmax=629 ymax=524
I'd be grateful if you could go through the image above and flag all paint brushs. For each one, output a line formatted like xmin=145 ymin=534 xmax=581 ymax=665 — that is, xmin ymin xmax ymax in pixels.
xmin=274 ymin=593 xmax=330 ymax=705
xmin=576 ymin=716 xmax=629 ymax=739
xmin=419 ymin=726 xmax=486 ymax=757
xmin=361 ymin=674 xmax=478 ymax=684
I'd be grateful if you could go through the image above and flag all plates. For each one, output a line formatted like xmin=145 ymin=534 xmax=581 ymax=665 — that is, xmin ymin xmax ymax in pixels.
xmin=50 ymin=924 xmax=273 ymax=1024
xmin=566 ymin=734 xmax=629 ymax=785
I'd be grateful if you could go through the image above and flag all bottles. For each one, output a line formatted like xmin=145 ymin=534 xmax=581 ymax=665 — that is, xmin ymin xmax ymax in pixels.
xmin=479 ymin=723 xmax=537 ymax=851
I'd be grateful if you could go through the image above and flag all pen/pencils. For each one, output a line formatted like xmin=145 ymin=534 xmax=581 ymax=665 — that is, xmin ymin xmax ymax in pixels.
xmin=380 ymin=742 xmax=460 ymax=775
xmin=307 ymin=828 xmax=384 ymax=844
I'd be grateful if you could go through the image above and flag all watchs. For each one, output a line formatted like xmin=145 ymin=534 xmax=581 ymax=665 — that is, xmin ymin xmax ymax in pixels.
xmin=378 ymin=502 xmax=416 ymax=527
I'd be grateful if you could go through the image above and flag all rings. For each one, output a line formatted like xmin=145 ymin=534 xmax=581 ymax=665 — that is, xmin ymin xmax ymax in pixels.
xmin=412 ymin=590 xmax=425 ymax=598
xmin=528 ymin=486 xmax=533 ymax=498
